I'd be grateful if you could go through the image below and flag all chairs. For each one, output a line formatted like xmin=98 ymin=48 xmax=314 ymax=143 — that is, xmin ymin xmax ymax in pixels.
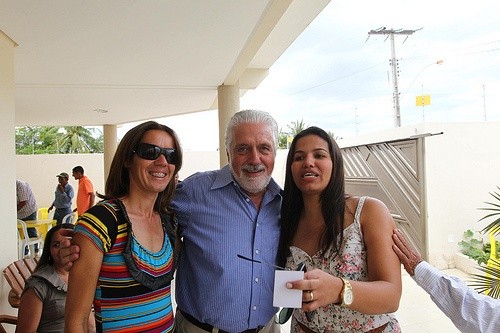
xmin=0 ymin=204 xmax=96 ymax=332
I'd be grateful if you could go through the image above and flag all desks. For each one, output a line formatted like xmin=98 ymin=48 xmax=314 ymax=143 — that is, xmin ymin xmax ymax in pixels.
xmin=18 ymin=220 xmax=58 ymax=241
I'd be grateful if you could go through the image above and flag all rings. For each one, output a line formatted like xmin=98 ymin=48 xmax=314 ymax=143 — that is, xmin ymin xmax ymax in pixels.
xmin=310 ymin=291 xmax=313 ymax=302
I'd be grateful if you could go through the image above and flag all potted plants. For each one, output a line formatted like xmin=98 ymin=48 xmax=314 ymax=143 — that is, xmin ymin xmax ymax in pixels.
xmin=456 ymin=229 xmax=499 ymax=273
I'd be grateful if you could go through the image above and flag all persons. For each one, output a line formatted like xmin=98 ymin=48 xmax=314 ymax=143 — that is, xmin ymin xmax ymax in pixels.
xmin=64 ymin=121 xmax=183 ymax=333
xmin=48 ymin=172 xmax=74 ymax=226
xmin=15 ymin=223 xmax=96 ymax=333
xmin=60 ymin=110 xmax=285 ymax=333
xmin=72 ymin=166 xmax=95 ymax=218
xmin=392 ymin=228 xmax=500 ymax=333
xmin=276 ymin=126 xmax=402 ymax=333
xmin=16 ymin=180 xmax=39 ymax=255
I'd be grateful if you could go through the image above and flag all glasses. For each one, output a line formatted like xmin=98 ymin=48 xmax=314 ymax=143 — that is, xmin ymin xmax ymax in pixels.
xmin=237 ymin=252 xmax=301 ymax=324
xmin=132 ymin=143 xmax=179 ymax=164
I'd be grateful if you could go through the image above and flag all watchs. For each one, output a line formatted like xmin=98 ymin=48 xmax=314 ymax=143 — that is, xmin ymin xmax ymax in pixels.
xmin=332 ymin=277 xmax=353 ymax=308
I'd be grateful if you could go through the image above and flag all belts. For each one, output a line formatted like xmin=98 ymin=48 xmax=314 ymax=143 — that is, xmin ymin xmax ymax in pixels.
xmin=176 ymin=305 xmax=265 ymax=333
xmin=56 ymin=208 xmax=71 ymax=211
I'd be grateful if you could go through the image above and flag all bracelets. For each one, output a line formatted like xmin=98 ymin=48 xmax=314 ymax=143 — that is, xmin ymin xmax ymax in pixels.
xmin=411 ymin=260 xmax=424 ymax=276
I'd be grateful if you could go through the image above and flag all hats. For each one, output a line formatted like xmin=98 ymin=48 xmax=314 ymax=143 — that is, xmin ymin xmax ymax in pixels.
xmin=56 ymin=172 xmax=69 ymax=179
